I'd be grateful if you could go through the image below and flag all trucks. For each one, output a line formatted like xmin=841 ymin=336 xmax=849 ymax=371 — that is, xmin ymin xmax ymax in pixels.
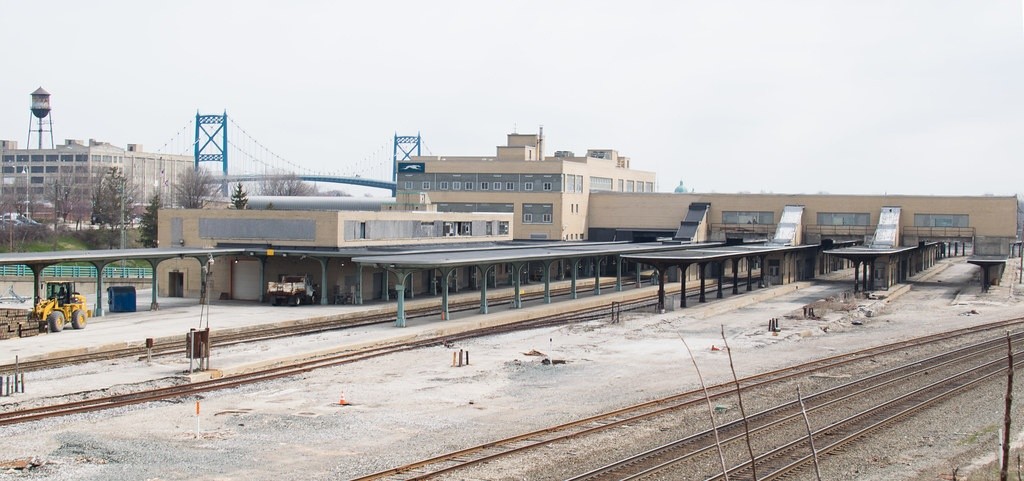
xmin=267 ymin=275 xmax=319 ymax=306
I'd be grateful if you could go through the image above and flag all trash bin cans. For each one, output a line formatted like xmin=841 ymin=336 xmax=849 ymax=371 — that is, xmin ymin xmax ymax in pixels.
xmin=107 ymin=286 xmax=136 ymax=313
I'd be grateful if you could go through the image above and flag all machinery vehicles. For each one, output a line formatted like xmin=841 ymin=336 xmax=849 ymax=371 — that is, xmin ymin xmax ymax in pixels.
xmin=26 ymin=279 xmax=93 ymax=331
